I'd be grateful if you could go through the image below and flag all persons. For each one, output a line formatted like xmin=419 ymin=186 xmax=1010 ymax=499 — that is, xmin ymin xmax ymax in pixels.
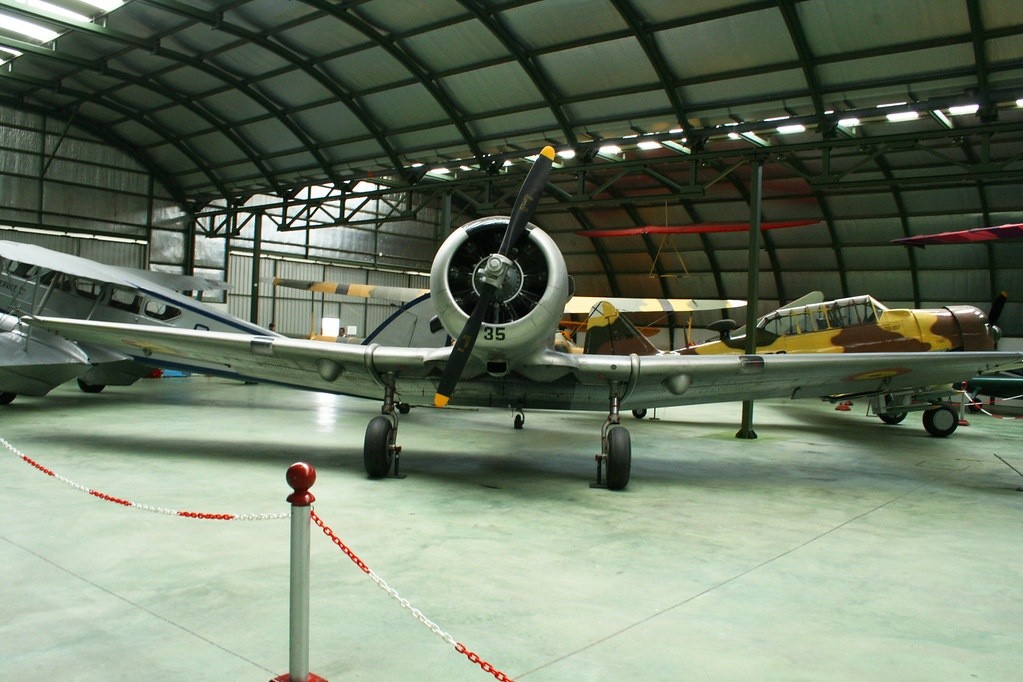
xmin=269 ymin=323 xmax=275 ymax=331
xmin=338 ymin=328 xmax=347 ymax=337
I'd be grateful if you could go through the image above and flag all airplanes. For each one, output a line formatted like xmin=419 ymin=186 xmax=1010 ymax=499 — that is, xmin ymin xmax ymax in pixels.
xmin=0 ymin=237 xmax=290 ymax=407
xmin=18 ymin=143 xmax=1022 ymax=490
xmin=547 ymin=293 xmax=1002 ymax=439
